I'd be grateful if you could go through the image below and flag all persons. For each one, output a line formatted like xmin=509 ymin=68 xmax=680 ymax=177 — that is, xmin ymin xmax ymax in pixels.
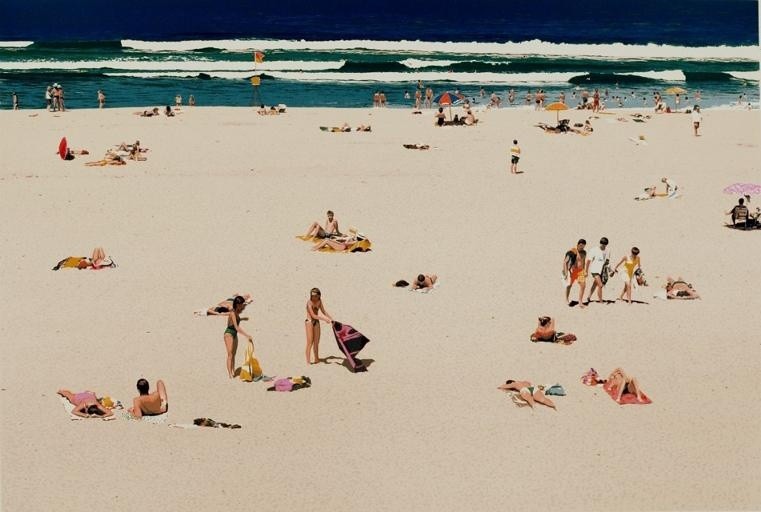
xmin=11 ymin=83 xmax=106 ymax=113
xmin=736 ymin=92 xmax=752 ymax=114
xmin=57 ymin=378 xmax=168 ymax=420
xmin=330 ymin=90 xmax=431 ymax=150
xmin=195 ymin=209 xmax=438 ymax=389
xmin=637 ymin=176 xmax=759 ymax=230
xmin=402 ymin=80 xmax=501 ymax=126
xmin=510 ymin=139 xmax=520 ymax=174
xmin=542 ymin=119 xmax=593 ymax=136
xmin=144 ymin=94 xmax=195 ymax=116
xmin=258 ymin=103 xmax=287 ymax=118
xmin=497 ymin=238 xmax=697 ymax=407
xmin=65 ymin=246 xmax=118 ymax=271
xmin=506 ymin=84 xmax=610 ymax=114
xmin=69 ymin=138 xmax=150 ymax=166
xmin=611 ymin=89 xmax=703 ymax=136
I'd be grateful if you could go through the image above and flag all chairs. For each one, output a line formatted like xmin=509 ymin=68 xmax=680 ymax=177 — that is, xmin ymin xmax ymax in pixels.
xmin=733 ymin=208 xmax=749 ymax=230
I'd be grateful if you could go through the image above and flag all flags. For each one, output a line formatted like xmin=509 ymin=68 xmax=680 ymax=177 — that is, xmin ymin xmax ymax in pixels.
xmin=255 ymin=52 xmax=265 ymax=64
